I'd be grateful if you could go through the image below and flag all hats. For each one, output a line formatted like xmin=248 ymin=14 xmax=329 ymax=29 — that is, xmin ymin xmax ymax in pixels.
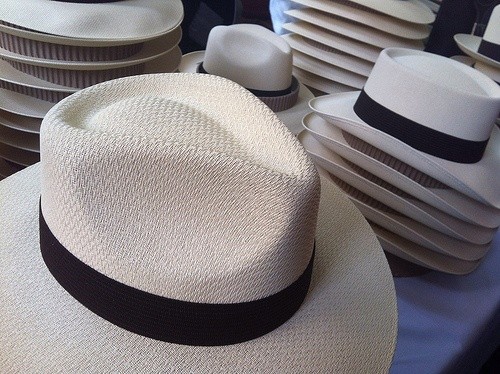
xmin=1 ymin=0 xmax=500 ymax=374
